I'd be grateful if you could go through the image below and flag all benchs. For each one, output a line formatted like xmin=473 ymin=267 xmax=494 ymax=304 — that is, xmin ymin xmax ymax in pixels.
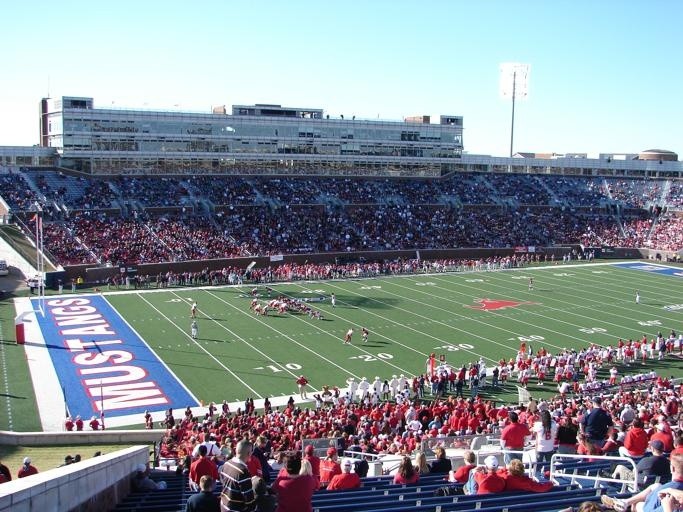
xmin=0 ymin=162 xmax=683 ymax=210
xmin=211 ymin=210 xmax=606 ymax=260
xmin=110 ymin=448 xmax=669 ymax=512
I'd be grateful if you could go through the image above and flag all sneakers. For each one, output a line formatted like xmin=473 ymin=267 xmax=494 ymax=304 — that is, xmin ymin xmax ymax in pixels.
xmin=601 ymin=469 xmax=614 ymax=479
xmin=601 ymin=494 xmax=629 ymax=512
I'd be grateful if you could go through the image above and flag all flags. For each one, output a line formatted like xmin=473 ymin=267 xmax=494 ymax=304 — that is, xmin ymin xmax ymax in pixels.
xmin=100 ymin=386 xmax=105 ymax=431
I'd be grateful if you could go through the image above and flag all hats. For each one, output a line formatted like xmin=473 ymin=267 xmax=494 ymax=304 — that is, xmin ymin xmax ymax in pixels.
xmin=136 ymin=464 xmax=147 ymax=473
xmin=65 ymin=415 xmax=98 ymax=421
xmin=305 ymin=444 xmax=314 ymax=452
xmin=341 ymin=459 xmax=352 ymax=468
xmin=24 ymin=457 xmax=32 ymax=464
xmin=648 ymin=439 xmax=665 ymax=451
xmin=349 ymin=374 xmax=405 ymax=381
xmin=327 ymin=447 xmax=337 ymax=456
xmin=484 ymin=455 xmax=499 ymax=468
xmin=654 ymin=424 xmax=665 ymax=431
xmin=590 ymin=396 xmax=602 ymax=404
xmin=65 ymin=455 xmax=74 ymax=460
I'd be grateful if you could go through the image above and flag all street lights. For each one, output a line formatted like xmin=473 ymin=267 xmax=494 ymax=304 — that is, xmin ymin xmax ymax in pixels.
xmin=499 ymin=59 xmax=531 ymax=158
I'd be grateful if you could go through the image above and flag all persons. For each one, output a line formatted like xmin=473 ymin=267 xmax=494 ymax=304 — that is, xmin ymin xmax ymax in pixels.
xmin=634 ymin=290 xmax=639 ymax=303
xmin=64 ymin=416 xmax=73 ymax=431
xmin=57 ymin=454 xmax=75 ymax=467
xmin=249 ymin=287 xmax=323 ymax=322
xmin=75 ymin=453 xmax=81 ymax=462
xmin=76 ymin=415 xmax=84 ymax=431
xmin=330 ymin=293 xmax=335 ymax=308
xmin=88 ymin=415 xmax=100 ymax=430
xmin=0 ymin=463 xmax=11 ymax=485
xmin=190 ymin=320 xmax=197 ymax=339
xmin=17 ymin=457 xmax=38 ymax=479
xmin=190 ymin=300 xmax=197 ymax=319
